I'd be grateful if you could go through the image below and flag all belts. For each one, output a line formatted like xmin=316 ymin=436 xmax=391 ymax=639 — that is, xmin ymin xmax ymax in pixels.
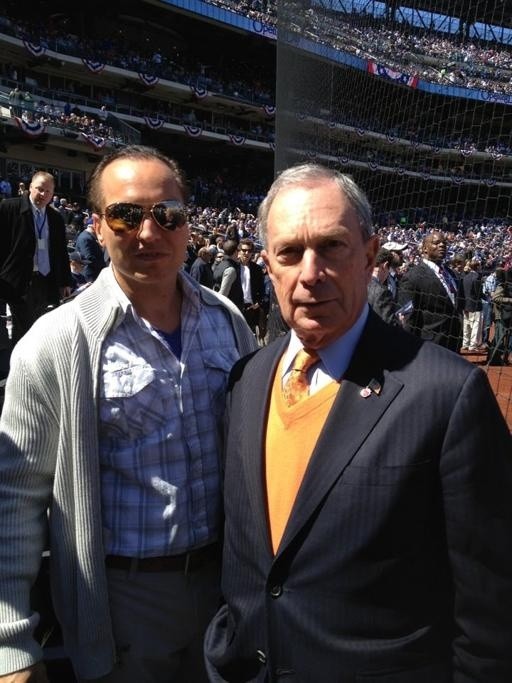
xmin=105 ymin=543 xmax=220 ymax=576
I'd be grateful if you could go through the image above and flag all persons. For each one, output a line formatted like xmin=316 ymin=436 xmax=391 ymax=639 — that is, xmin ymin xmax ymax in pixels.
xmin=199 ymin=168 xmax=511 ymax=683
xmin=2 ymin=147 xmax=263 ymax=682
xmin=2 ymin=3 xmax=511 ymax=364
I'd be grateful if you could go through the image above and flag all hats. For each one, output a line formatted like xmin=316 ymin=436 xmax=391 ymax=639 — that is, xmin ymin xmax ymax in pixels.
xmin=86 ymin=218 xmax=93 ymax=224
xmin=382 ymin=242 xmax=408 ymax=251
xmin=69 ymin=252 xmax=92 ymax=265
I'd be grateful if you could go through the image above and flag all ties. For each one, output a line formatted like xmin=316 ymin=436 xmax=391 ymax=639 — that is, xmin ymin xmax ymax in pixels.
xmin=36 ymin=210 xmax=50 ymax=277
xmin=439 ymin=268 xmax=456 ymax=293
xmin=283 ymin=349 xmax=320 ymax=407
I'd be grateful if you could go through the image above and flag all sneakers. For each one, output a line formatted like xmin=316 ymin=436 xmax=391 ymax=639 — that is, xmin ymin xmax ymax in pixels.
xmin=462 ymin=346 xmax=479 ymax=352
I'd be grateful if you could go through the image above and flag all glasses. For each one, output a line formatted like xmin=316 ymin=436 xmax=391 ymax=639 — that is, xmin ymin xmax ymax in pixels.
xmin=241 ymin=249 xmax=252 ymax=253
xmin=96 ymin=201 xmax=192 ymax=235
xmin=217 ymin=256 xmax=223 ymax=258
